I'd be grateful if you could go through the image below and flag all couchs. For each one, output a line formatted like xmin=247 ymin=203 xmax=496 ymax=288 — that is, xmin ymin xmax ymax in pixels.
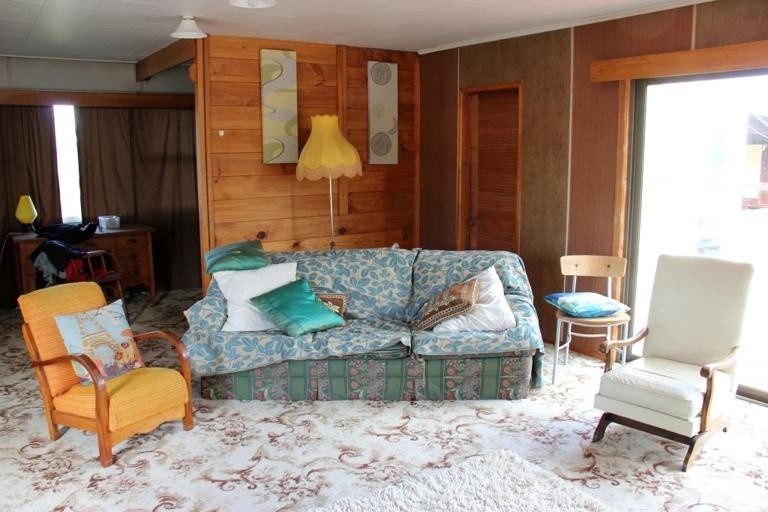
xmin=176 ymin=243 xmax=546 ymax=409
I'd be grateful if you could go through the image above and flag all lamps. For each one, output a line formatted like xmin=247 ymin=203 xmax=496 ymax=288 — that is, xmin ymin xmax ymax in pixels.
xmin=166 ymin=15 xmax=209 ymax=40
xmin=294 ymin=111 xmax=365 ymax=250
xmin=12 ymin=193 xmax=39 ymax=236
xmin=226 ymin=0 xmax=279 ymax=9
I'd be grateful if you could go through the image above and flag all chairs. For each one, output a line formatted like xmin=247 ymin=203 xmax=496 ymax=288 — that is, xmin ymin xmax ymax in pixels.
xmin=15 ymin=277 xmax=195 ymax=469
xmin=588 ymin=248 xmax=756 ymax=476
xmin=550 ymin=253 xmax=633 ymax=389
xmin=37 ymin=238 xmax=126 ymax=309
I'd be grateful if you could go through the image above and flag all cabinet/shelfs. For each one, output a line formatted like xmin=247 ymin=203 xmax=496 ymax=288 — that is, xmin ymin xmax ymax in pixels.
xmin=9 ymin=222 xmax=158 ymax=309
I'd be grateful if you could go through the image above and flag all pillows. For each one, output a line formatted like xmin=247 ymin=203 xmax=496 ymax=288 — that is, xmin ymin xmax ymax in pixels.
xmin=247 ymin=276 xmax=347 ymax=339
xmin=52 ymin=298 xmax=146 ymax=389
xmin=539 ymin=289 xmax=631 ymax=319
xmin=210 ymin=260 xmax=300 ymax=333
xmin=431 ymin=263 xmax=517 ymax=334
xmin=314 ymin=287 xmax=349 ymax=317
xmin=202 ymin=238 xmax=270 ymax=275
xmin=407 ymin=276 xmax=480 ymax=333
